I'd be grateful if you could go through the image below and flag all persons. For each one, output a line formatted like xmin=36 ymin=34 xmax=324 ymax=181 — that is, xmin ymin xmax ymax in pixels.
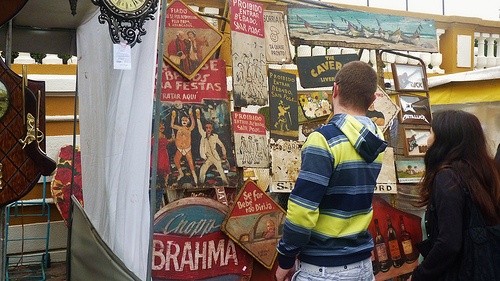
xmin=406 ymin=109 xmax=500 ymax=281
xmin=274 ymin=61 xmax=388 ymax=281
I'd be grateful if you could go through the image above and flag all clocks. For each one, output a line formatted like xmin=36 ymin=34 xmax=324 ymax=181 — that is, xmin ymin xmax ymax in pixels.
xmin=91 ymin=0 xmax=160 ymax=48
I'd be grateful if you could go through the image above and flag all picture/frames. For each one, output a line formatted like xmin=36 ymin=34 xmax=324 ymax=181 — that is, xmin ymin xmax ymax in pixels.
xmin=0 ymin=79 xmax=11 ymax=121
xmin=399 ymin=124 xmax=431 ymax=158
xmin=395 ymin=94 xmax=432 ymax=125
xmin=391 ymin=62 xmax=429 ymax=92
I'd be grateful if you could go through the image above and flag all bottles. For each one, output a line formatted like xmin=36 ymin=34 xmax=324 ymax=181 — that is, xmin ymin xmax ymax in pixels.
xmin=399 ymin=215 xmax=419 ymax=263
xmin=371 ymin=248 xmax=377 ymax=276
xmin=374 ymin=213 xmax=404 ymax=273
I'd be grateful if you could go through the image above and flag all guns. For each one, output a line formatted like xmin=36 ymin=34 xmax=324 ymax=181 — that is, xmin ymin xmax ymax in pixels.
xmin=17 ymin=64 xmax=58 ymax=177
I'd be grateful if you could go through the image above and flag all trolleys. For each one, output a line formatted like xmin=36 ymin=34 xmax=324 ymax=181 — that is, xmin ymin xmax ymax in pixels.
xmin=3 ymin=200 xmax=53 ymax=281
